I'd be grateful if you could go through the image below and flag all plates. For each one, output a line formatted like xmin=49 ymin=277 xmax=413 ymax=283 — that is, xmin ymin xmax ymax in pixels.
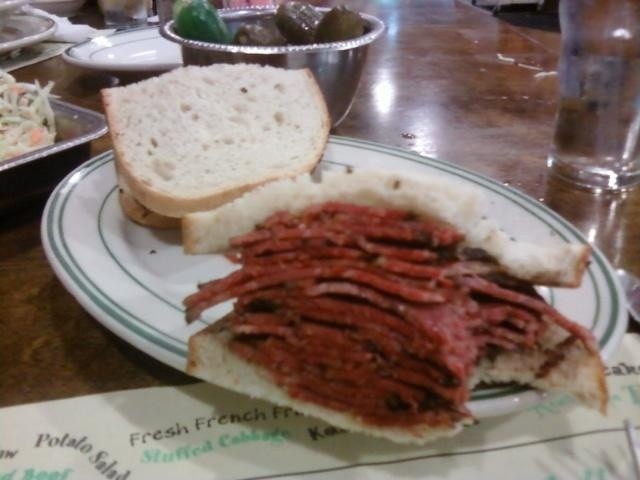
xmin=39 ymin=132 xmax=630 ymax=430
xmin=63 ymin=25 xmax=185 ymax=71
xmin=0 ymin=14 xmax=56 ymax=53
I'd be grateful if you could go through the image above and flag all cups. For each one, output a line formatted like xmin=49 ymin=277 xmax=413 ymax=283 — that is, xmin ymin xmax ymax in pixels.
xmin=547 ymin=0 xmax=637 ymax=191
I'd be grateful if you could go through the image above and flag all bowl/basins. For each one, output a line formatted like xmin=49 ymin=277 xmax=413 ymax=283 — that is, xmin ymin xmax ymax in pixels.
xmin=160 ymin=6 xmax=384 ymax=128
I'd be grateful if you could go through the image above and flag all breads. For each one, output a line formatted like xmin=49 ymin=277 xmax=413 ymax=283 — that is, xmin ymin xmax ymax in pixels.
xmin=99 ymin=62 xmax=609 ymax=446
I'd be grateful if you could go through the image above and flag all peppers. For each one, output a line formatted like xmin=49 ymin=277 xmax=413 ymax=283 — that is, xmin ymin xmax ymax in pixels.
xmin=173 ymin=0 xmax=365 ymax=46
